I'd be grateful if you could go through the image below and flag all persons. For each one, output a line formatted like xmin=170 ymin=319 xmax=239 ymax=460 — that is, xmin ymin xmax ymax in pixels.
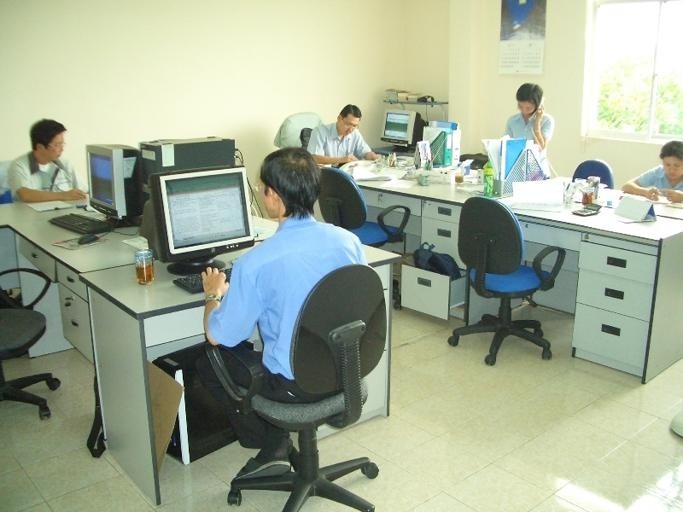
xmin=306 ymin=104 xmax=381 ymax=170
xmin=620 ymin=140 xmax=683 ymax=205
xmin=8 ymin=119 xmax=86 ymax=203
xmin=503 ymin=82 xmax=554 ymax=156
xmin=200 ymin=147 xmax=368 ymax=483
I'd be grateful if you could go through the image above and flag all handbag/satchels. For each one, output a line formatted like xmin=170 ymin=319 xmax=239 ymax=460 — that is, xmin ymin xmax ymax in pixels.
xmin=413 ymin=242 xmax=461 ymax=280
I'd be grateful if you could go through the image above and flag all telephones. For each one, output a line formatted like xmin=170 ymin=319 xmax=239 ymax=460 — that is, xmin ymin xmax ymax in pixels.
xmin=530 ymin=95 xmax=545 ymax=119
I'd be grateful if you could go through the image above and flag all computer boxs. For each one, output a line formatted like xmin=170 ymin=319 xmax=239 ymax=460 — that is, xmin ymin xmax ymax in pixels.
xmin=138 ymin=135 xmax=236 ymax=216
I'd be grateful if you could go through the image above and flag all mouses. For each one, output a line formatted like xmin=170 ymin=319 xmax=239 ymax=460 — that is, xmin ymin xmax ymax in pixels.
xmin=77 ymin=234 xmax=99 ymax=244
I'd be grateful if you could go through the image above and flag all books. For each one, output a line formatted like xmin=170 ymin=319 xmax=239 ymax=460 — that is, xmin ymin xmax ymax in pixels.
xmin=482 ymin=134 xmax=527 ymax=182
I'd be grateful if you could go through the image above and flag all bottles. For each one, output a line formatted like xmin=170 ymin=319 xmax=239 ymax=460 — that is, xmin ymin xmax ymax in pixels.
xmin=606 ymin=189 xmax=613 ymax=208
xmin=476 ymin=169 xmax=482 ymax=184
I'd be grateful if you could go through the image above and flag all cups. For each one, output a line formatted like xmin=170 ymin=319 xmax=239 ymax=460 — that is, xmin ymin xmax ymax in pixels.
xmin=562 ymin=182 xmax=576 ymax=207
xmin=454 ymin=170 xmax=464 ymax=184
xmin=587 ymin=176 xmax=600 ymax=200
xmin=417 ymin=172 xmax=429 ymax=185
xmin=574 ymin=178 xmax=587 ymax=202
xmin=135 ymin=249 xmax=153 ymax=285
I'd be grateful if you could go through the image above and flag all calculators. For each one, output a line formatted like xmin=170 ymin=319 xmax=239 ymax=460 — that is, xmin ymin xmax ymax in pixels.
xmin=572 ymin=203 xmax=601 ymax=216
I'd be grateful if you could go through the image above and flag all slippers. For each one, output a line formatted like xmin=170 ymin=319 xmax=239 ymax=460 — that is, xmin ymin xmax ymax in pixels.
xmin=233 ymin=457 xmax=291 ymax=480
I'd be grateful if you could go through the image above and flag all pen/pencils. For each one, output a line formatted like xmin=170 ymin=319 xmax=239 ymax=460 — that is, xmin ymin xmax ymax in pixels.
xmin=652 ymin=193 xmax=656 ymax=196
xmin=388 ymin=152 xmax=397 ymax=167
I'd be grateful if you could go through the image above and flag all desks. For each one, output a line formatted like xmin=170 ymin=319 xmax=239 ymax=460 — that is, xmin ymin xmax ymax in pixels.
xmin=0 ymin=193 xmax=278 ymax=365
xmin=465 ymin=189 xmax=683 ymax=385
xmin=79 ymin=239 xmax=402 ymax=505
xmin=373 ymin=100 xmax=448 ymax=156
xmin=353 ymin=166 xmax=513 ymax=323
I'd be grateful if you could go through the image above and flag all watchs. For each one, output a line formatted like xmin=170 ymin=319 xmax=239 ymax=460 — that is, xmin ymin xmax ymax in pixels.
xmin=204 ymin=293 xmax=221 ymax=303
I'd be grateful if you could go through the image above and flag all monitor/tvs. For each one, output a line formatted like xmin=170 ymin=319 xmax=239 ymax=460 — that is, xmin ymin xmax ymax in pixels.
xmin=380 ymin=109 xmax=425 ymax=151
xmin=139 ymin=164 xmax=255 ymax=275
xmin=86 ymin=143 xmax=139 ymax=227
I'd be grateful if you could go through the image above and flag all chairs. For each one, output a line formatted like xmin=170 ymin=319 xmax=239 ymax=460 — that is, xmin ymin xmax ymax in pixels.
xmin=572 ymin=159 xmax=614 ymax=189
xmin=0 ymin=191 xmax=13 ymax=204
xmin=273 ymin=112 xmax=322 ymax=152
xmin=0 ymin=267 xmax=61 ymax=420
xmin=448 ymin=197 xmax=566 ymax=365
xmin=203 ymin=265 xmax=387 ymax=512
xmin=318 ymin=166 xmax=410 ymax=310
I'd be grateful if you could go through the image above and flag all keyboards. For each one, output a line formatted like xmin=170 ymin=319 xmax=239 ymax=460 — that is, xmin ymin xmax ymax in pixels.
xmin=48 ymin=213 xmax=111 ymax=234
xmin=173 ymin=267 xmax=235 ymax=295
